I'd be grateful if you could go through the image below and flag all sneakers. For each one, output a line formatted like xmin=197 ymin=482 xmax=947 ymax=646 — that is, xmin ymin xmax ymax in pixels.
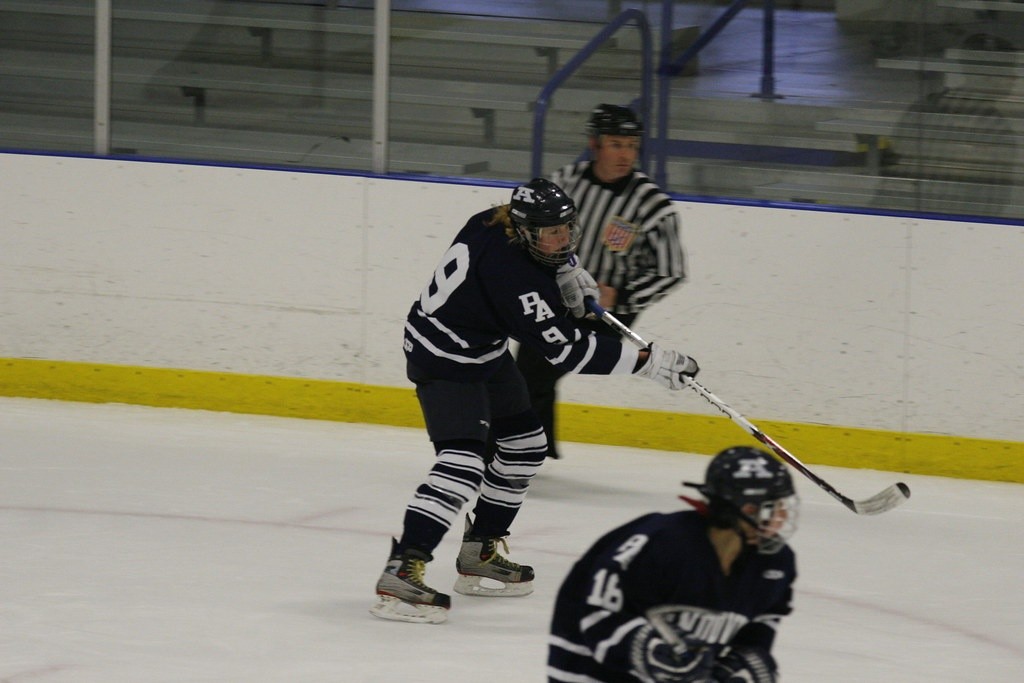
xmin=369 ymin=536 xmax=451 ymax=624
xmin=452 ymin=512 xmax=535 ymax=596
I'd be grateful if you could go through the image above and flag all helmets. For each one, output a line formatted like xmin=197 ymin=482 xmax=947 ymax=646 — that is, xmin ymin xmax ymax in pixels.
xmin=705 ymin=445 xmax=796 ymax=521
xmin=584 ymin=103 xmax=647 ymax=136
xmin=508 ymin=178 xmax=578 ymax=242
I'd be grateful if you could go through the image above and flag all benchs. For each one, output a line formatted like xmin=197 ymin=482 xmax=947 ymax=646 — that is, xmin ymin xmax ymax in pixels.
xmin=0 ymin=0 xmax=1024 ymax=220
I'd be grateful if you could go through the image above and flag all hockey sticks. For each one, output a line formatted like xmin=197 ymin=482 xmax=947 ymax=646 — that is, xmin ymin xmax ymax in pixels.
xmin=586 ymin=292 xmax=910 ymax=518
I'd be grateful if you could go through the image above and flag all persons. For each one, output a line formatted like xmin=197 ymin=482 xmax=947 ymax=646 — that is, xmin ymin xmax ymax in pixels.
xmin=546 ymin=103 xmax=690 ymax=342
xmin=546 ymin=446 xmax=802 ymax=683
xmin=369 ymin=174 xmax=700 ymax=623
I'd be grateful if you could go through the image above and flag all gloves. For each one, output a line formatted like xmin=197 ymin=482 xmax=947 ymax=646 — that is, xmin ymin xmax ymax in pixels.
xmin=646 ymin=637 xmax=714 ymax=683
xmin=556 ymin=254 xmax=600 ymax=319
xmin=631 ymin=341 xmax=701 ymax=391
